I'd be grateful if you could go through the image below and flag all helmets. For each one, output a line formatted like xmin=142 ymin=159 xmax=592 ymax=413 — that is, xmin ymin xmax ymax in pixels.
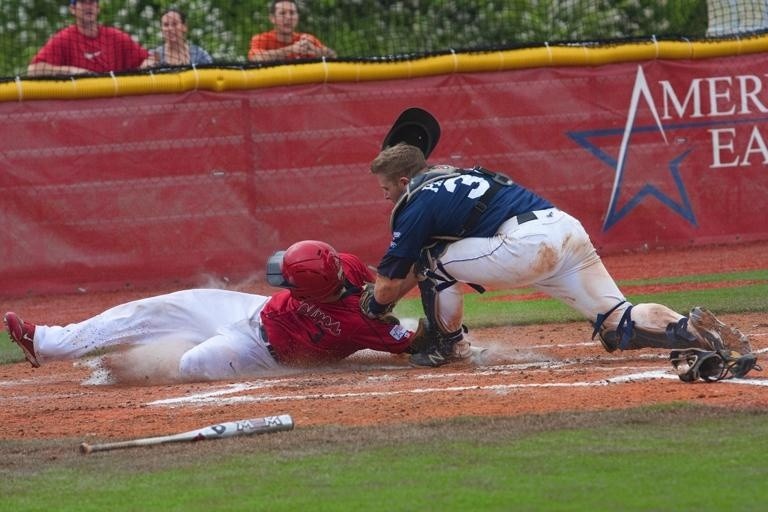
xmin=382 ymin=108 xmax=440 ymax=159
xmin=265 ymin=241 xmax=346 ymax=304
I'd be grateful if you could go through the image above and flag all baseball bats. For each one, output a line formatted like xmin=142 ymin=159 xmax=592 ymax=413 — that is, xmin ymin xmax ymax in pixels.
xmin=79 ymin=414 xmax=294 ymax=454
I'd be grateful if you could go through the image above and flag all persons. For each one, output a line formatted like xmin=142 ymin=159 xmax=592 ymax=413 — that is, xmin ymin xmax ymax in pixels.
xmin=3 ymin=240 xmax=427 ymax=383
xmin=360 ymin=142 xmax=751 ymax=374
xmin=147 ymin=10 xmax=212 ymax=66
xmin=249 ymin=0 xmax=338 ymax=61
xmin=26 ymin=0 xmax=158 ymax=76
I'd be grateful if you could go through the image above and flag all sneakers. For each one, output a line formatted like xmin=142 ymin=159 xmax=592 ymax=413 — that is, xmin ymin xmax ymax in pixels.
xmin=5 ymin=312 xmax=41 ymax=371
xmin=410 ymin=338 xmax=473 ymax=368
xmin=687 ymin=305 xmax=752 ymax=355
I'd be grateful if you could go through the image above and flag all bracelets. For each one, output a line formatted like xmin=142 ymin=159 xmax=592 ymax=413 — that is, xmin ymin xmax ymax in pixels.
xmin=367 ymin=297 xmax=393 ymax=316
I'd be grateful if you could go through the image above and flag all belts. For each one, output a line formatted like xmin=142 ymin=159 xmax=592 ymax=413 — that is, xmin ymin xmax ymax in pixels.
xmin=260 ymin=327 xmax=280 ymax=364
xmin=516 ymin=211 xmax=536 ymax=224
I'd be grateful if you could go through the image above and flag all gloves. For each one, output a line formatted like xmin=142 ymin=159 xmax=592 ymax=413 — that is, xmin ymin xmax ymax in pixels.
xmin=359 ymin=285 xmax=396 ymax=320
